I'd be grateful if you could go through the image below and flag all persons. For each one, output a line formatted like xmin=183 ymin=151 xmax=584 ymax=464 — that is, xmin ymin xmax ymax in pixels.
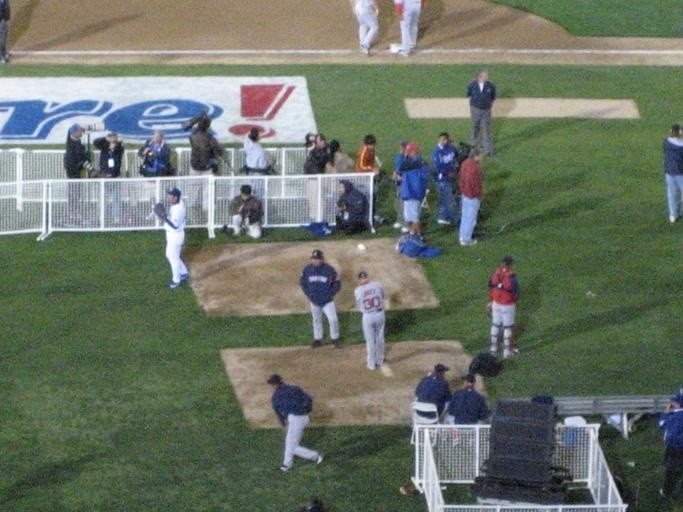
xmin=299 ymin=249 xmax=342 ymax=348
xmin=136 ymin=129 xmax=170 ymax=222
xmin=392 ymin=1 xmax=425 ymax=56
xmin=464 ymin=68 xmax=496 ymax=159
xmin=657 ymin=390 xmax=683 ymax=501
xmin=61 ymin=123 xmax=97 ymax=226
xmin=188 ymin=117 xmax=221 ymax=211
xmin=392 ymin=131 xmax=485 ymax=249
xmin=305 ymin=133 xmax=354 ymax=192
xmin=350 ymin=269 xmax=387 ymax=374
xmin=350 ymin=0 xmax=380 ymax=57
xmin=0 ymin=0 xmax=12 ymax=64
xmin=243 ymin=128 xmax=270 ymax=187
xmin=152 ymin=186 xmax=189 ymax=289
xmin=661 ymin=123 xmax=683 ymax=226
xmin=336 ymin=180 xmax=368 ymax=235
xmin=413 ymin=362 xmax=453 ymax=421
xmin=356 ymin=134 xmax=381 ymax=218
xmin=230 ymin=185 xmax=262 ymax=238
xmin=265 ymin=373 xmax=328 ymax=474
xmin=486 ymin=256 xmax=521 ymax=361
xmin=92 ymin=132 xmax=124 ymax=224
xmin=446 ymin=374 xmax=489 ymax=427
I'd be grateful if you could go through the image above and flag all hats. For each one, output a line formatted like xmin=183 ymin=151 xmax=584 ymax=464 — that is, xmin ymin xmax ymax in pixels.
xmin=461 ymin=375 xmax=474 ymax=382
xmin=266 ymin=375 xmax=280 ymax=384
xmin=310 ymin=250 xmax=321 ymax=259
xmin=434 ymin=364 xmax=448 ymax=371
xmin=166 ymin=188 xmax=180 ymax=196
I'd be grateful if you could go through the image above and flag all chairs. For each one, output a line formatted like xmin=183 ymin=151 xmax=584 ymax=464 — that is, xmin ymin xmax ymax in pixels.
xmin=409 ymin=402 xmax=441 ymax=447
xmin=421 ymin=188 xmax=431 ymax=216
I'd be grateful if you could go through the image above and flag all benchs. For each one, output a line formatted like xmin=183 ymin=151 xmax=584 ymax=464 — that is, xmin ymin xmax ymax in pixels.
xmin=554 ymin=394 xmax=674 ymax=440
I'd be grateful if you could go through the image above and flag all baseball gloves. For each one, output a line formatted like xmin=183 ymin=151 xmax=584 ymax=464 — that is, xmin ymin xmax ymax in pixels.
xmin=487 ymin=301 xmax=494 ymax=316
xmin=154 ymin=202 xmax=166 ymax=218
